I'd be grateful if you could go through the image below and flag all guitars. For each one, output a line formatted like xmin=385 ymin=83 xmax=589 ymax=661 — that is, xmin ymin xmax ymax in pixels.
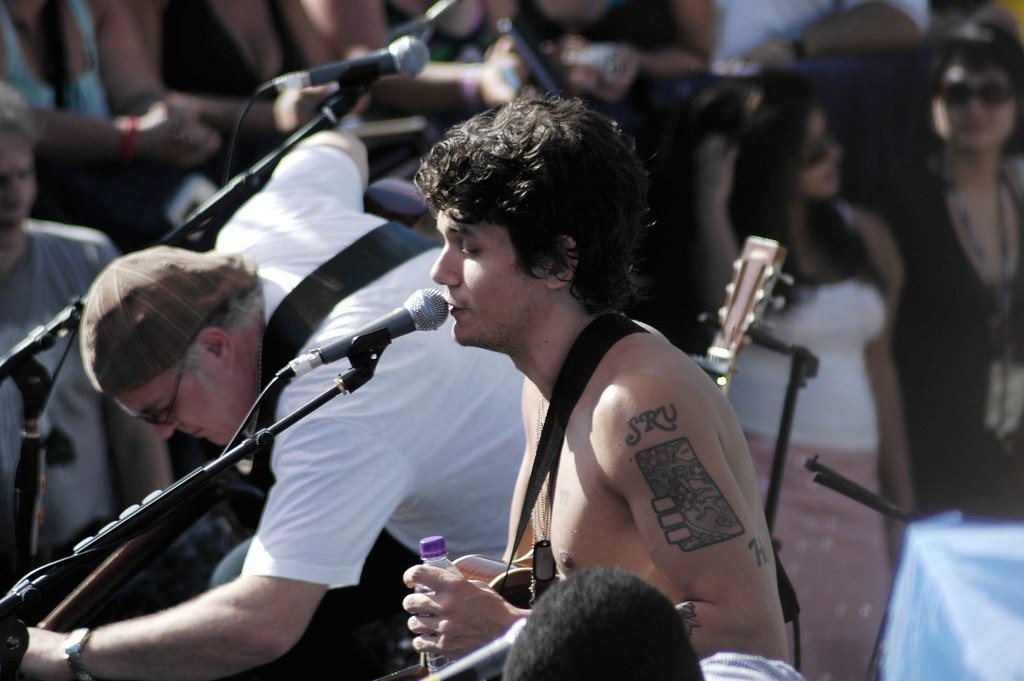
xmin=694 ymin=232 xmax=796 ymax=397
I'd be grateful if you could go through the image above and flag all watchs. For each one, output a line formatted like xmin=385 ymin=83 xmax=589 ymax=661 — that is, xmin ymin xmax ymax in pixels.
xmin=64 ymin=627 xmax=93 ymax=681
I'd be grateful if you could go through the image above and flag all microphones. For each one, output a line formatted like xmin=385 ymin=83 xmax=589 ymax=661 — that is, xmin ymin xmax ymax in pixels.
xmin=421 ymin=618 xmax=529 ymax=681
xmin=255 ymin=36 xmax=430 ymax=99
xmin=277 ymin=288 xmax=448 ymax=382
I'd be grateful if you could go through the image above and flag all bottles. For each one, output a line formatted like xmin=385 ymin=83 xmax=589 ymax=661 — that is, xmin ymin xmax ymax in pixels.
xmin=415 ymin=536 xmax=464 ymax=677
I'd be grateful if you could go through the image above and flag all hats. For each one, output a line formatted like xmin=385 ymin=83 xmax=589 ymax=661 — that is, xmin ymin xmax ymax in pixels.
xmin=80 ymin=245 xmax=256 ymax=396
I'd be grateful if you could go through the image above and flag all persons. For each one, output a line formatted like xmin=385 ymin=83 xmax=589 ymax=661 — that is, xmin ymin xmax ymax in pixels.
xmin=502 ymin=564 xmax=805 ymax=681
xmin=18 ymin=129 xmax=550 ymax=681
xmin=0 ymin=0 xmax=1024 ymax=625
xmin=403 ymin=89 xmax=790 ymax=681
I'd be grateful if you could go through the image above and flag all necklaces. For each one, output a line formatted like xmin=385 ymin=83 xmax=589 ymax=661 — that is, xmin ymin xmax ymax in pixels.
xmin=528 ymin=392 xmax=563 ymax=608
xmin=251 ymin=328 xmax=264 ymax=435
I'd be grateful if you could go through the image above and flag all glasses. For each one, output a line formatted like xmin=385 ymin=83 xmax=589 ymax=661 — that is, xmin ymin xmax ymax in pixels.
xmin=797 ymin=119 xmax=838 ymax=171
xmin=145 ymin=350 xmax=186 ymax=427
xmin=942 ymin=82 xmax=1014 ymax=104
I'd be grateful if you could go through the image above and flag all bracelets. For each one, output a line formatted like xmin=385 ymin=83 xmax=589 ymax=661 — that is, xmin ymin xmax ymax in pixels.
xmin=117 ymin=116 xmax=136 ymax=151
